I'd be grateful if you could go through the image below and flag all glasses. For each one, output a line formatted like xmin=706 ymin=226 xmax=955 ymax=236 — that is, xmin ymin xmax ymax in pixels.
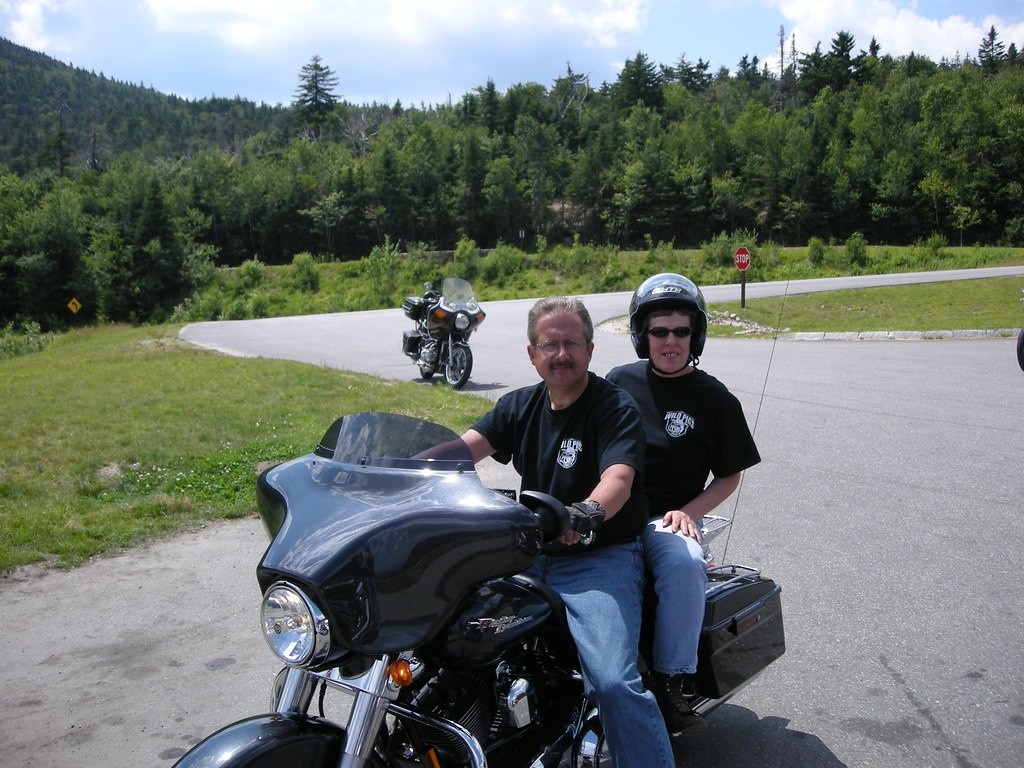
xmin=534 ymin=339 xmax=587 ymax=354
xmin=647 ymin=326 xmax=694 ymax=337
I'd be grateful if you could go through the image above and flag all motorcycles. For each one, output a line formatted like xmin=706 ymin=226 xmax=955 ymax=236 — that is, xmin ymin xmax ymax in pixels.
xmin=402 ymin=278 xmax=486 ymax=389
xmin=171 ymin=412 xmax=786 ymax=768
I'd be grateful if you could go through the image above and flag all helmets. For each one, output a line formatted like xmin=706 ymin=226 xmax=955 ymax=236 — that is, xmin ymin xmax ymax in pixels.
xmin=629 ymin=273 xmax=707 ymax=359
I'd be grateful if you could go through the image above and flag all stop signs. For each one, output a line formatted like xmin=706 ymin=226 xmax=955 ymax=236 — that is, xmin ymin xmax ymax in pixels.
xmin=735 ymin=247 xmax=750 ymax=270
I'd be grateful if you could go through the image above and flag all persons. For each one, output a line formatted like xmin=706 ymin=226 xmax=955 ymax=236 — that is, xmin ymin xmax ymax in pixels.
xmin=406 ymin=297 xmax=676 ymax=768
xmin=604 ymin=272 xmax=761 ymax=734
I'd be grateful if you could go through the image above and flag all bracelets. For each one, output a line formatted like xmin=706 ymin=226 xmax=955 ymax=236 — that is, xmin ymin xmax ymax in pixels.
xmin=584 ymin=500 xmax=606 ymax=512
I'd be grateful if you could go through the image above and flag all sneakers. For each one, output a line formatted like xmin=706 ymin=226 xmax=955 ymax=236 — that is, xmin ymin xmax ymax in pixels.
xmin=654 ymin=677 xmax=708 ymax=733
